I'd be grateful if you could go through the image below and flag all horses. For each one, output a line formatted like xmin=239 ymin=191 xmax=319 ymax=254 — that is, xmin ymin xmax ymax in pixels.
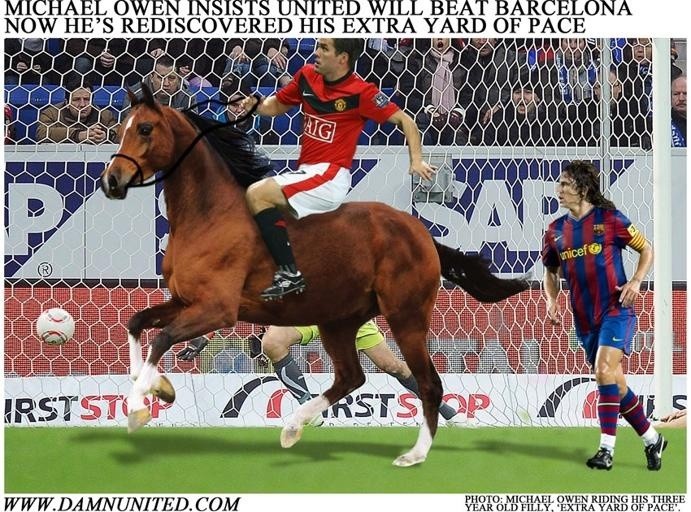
xmin=102 ymin=83 xmax=533 ymax=469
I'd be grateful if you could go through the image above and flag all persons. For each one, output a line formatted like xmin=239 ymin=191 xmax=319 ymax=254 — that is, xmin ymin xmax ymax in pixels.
xmin=237 ymin=38 xmax=440 ymax=299
xmin=4 ymin=37 xmax=687 ymax=150
xmin=542 ymin=160 xmax=668 ymax=470
xmin=178 ymin=322 xmax=458 ymax=426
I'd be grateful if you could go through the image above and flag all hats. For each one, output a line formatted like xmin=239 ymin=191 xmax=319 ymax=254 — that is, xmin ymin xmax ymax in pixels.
xmin=513 ymin=71 xmax=542 ymax=99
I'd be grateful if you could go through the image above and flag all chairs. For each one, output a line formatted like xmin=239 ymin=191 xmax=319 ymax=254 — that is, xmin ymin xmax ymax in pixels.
xmin=6 ymin=39 xmax=622 ymax=145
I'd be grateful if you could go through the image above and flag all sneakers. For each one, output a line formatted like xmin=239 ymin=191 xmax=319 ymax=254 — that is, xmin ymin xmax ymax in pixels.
xmin=643 ymin=433 xmax=668 ymax=470
xmin=259 ymin=270 xmax=306 ymax=302
xmin=586 ymin=447 xmax=616 ymax=470
xmin=445 ymin=413 xmax=469 ymax=428
xmin=303 ymin=412 xmax=325 ymax=427
